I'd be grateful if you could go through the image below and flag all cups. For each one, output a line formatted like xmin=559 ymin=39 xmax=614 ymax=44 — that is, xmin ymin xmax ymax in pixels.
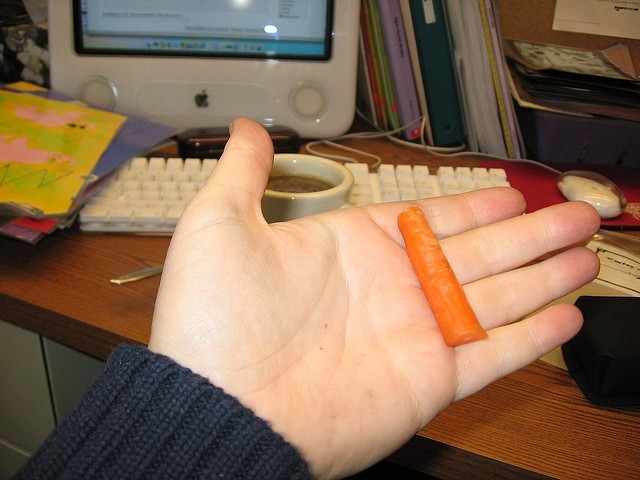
xmin=261 ymin=152 xmax=355 ymax=219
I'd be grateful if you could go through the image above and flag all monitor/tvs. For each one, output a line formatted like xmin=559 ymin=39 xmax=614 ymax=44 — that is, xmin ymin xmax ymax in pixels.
xmin=47 ymin=0 xmax=363 ymax=140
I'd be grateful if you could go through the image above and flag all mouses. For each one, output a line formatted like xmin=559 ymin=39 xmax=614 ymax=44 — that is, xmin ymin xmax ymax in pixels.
xmin=558 ymin=167 xmax=628 ymax=219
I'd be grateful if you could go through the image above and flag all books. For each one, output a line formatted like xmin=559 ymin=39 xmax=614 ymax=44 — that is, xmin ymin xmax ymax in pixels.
xmin=359 ymin=1 xmax=527 ymax=160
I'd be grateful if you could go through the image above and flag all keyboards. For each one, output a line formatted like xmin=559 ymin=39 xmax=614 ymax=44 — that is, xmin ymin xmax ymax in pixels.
xmin=77 ymin=155 xmax=514 ymax=236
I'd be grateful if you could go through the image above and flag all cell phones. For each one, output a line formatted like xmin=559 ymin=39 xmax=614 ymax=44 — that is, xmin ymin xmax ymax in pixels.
xmin=176 ymin=127 xmax=302 ymax=155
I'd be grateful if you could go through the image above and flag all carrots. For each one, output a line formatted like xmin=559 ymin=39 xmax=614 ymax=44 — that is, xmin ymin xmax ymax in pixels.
xmin=397 ymin=206 xmax=490 ymax=347
